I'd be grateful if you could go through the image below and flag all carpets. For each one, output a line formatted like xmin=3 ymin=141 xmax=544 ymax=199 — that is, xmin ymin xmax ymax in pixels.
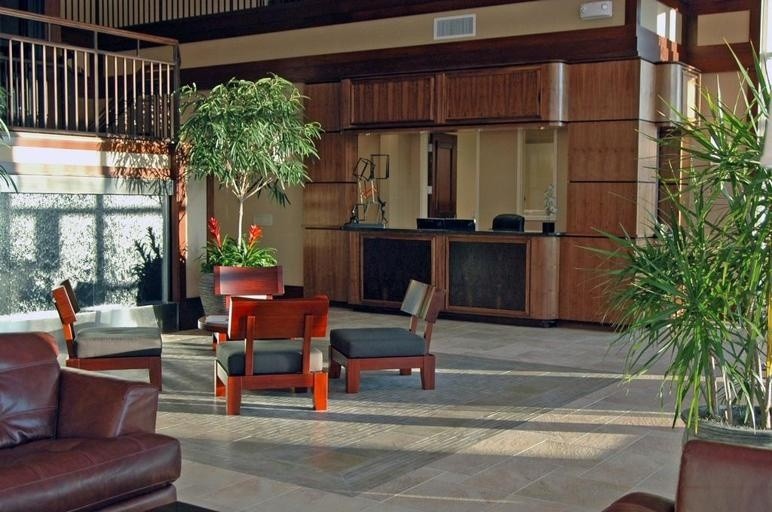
xmin=58 ymin=332 xmax=634 ymax=498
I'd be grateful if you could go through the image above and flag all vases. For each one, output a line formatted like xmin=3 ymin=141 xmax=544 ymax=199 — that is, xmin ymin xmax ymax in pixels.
xmin=197 ymin=273 xmax=228 ymax=316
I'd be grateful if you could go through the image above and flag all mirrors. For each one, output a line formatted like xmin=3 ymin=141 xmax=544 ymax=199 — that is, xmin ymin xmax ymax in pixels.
xmin=358 ymin=130 xmax=557 ymax=232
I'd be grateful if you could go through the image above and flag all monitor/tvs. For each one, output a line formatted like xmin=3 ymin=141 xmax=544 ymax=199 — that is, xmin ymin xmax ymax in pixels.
xmin=417 ymin=218 xmax=445 ymax=229
xmin=445 ymin=219 xmax=475 ymax=231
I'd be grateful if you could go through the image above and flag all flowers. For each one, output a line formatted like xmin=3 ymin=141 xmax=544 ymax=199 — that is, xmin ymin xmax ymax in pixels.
xmin=193 ymin=215 xmax=279 ymax=273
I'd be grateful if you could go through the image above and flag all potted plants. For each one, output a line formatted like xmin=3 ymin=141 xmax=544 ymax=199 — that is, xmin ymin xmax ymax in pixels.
xmin=542 ymin=184 xmax=555 ymax=233
xmin=571 ymin=37 xmax=772 ymax=445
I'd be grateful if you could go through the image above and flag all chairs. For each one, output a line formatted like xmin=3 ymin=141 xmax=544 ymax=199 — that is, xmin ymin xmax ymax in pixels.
xmin=0 ymin=332 xmax=182 ymax=512
xmin=50 ymin=279 xmax=165 ymax=393
xmin=492 ymin=214 xmax=525 ymax=232
xmin=196 ymin=264 xmax=446 ymax=415
